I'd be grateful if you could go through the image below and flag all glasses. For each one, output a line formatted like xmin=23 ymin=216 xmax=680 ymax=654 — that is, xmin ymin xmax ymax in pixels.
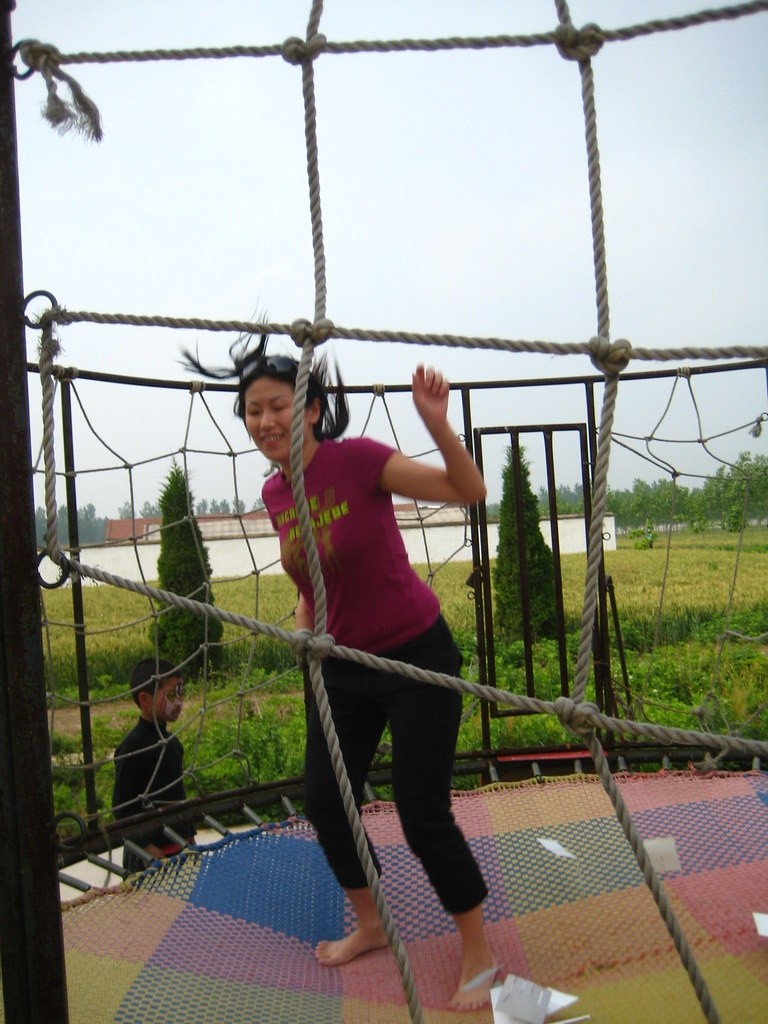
xmin=240 ymin=354 xmax=300 ymax=379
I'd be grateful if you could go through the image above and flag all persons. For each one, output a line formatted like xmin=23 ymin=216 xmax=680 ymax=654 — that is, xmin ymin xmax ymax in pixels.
xmin=111 ymin=657 xmax=199 ymax=882
xmin=181 ymin=321 xmax=501 ymax=1010
